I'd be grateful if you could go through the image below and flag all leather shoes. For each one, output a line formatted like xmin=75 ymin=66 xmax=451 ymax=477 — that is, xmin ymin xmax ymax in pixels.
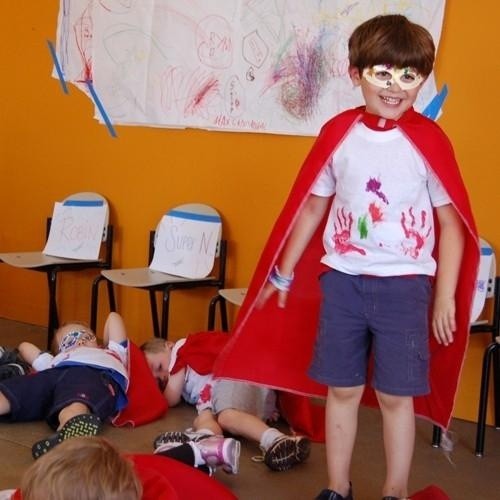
xmin=315 ymin=481 xmax=352 ymax=500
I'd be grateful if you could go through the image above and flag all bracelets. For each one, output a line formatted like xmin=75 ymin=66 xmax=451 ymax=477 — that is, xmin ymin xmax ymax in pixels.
xmin=267 ymin=265 xmax=295 ymax=293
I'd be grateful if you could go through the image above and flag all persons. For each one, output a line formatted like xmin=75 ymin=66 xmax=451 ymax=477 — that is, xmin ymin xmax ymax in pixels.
xmin=18 ymin=434 xmax=243 ymax=500
xmin=137 ymin=336 xmax=313 ymax=474
xmin=256 ymin=15 xmax=468 ymax=499
xmin=0 ymin=312 xmax=130 ymax=461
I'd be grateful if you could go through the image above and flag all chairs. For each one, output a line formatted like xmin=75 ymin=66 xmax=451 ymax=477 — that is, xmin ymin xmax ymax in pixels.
xmin=1 ymin=190 xmax=227 ymax=355
xmin=432 ymin=236 xmax=498 ymax=462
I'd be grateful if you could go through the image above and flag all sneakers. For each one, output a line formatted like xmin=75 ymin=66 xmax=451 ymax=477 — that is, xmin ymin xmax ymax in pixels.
xmin=153 ymin=432 xmax=215 ymax=450
xmin=32 ymin=414 xmax=101 ymax=459
xmin=194 ymin=438 xmax=241 ymax=476
xmin=251 ymin=436 xmax=310 ymax=472
